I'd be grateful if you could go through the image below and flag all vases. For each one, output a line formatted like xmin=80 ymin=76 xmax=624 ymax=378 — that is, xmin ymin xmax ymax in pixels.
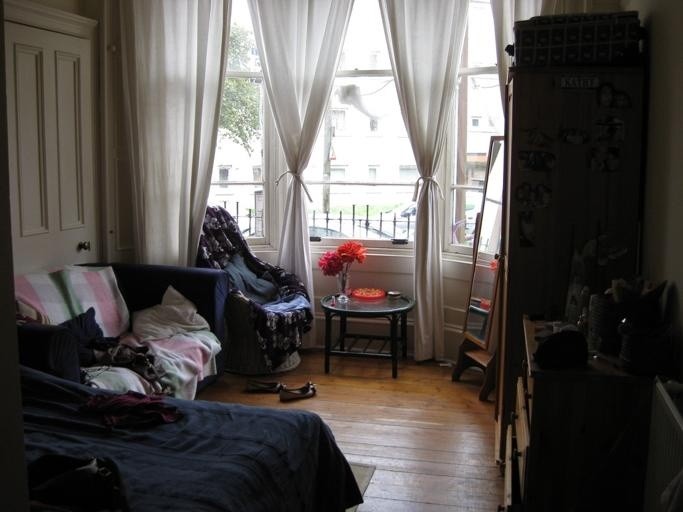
xmin=337 ymin=274 xmax=351 ymax=296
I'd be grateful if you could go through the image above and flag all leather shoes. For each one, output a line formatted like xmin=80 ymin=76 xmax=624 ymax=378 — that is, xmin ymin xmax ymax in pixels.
xmin=280 ymin=381 xmax=315 ymax=401
xmin=247 ymin=379 xmax=281 ymax=390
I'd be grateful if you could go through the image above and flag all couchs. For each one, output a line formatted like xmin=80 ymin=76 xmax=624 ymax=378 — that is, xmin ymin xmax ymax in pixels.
xmin=14 ymin=263 xmax=228 ymax=402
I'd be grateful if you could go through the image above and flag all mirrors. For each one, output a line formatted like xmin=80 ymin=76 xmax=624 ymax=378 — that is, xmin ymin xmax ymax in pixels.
xmin=451 ymin=135 xmax=504 ymax=402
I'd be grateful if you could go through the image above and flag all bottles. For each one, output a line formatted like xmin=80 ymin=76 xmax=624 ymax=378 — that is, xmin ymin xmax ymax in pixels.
xmin=330 ymin=296 xmax=335 ymax=308
xmin=552 ymin=322 xmax=560 ymax=333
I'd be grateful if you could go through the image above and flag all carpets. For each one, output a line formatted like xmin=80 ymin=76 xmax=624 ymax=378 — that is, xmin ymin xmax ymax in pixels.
xmin=345 ymin=460 xmax=375 ymax=512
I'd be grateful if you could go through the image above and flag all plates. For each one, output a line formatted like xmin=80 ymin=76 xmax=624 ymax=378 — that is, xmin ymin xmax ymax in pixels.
xmin=388 ymin=292 xmax=401 ymax=297
xmin=352 ymin=288 xmax=387 ymax=301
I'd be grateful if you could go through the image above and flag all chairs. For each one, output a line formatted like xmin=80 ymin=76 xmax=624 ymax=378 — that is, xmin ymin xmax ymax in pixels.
xmin=197 ymin=206 xmax=313 ymax=374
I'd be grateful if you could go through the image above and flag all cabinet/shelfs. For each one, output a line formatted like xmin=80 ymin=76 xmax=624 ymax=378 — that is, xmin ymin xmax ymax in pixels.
xmin=496 ymin=315 xmax=649 ymax=511
xmin=495 ymin=12 xmax=645 ymax=469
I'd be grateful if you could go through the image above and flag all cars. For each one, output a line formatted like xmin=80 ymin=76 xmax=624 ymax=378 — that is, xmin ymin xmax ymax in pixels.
xmin=373 ymin=201 xmax=472 ymax=231
xmin=240 ymin=220 xmax=395 ymax=240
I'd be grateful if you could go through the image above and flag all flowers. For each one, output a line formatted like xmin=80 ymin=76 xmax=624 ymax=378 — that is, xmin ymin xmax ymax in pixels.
xmin=318 ymin=242 xmax=368 ymax=292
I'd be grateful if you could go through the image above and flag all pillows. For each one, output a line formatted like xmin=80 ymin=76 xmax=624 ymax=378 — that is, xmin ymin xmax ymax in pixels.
xmin=15 ymin=269 xmax=72 ymax=325
xmin=62 ymin=265 xmax=131 ymax=338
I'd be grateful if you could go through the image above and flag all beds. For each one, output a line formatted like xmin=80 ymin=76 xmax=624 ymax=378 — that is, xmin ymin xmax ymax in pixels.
xmin=24 ymin=394 xmax=364 ymax=512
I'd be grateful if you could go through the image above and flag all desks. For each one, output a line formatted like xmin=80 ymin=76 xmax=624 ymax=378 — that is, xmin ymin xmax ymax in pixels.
xmin=321 ymin=293 xmax=415 ymax=378
xmin=469 ymin=298 xmax=492 ymax=340
